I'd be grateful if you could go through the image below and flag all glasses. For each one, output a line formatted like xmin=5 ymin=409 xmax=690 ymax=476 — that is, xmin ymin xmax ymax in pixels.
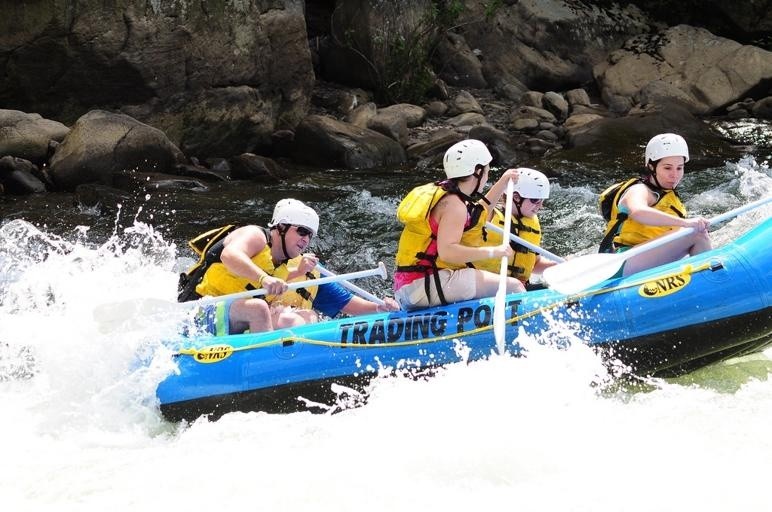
xmin=288 ymin=227 xmax=313 ymax=240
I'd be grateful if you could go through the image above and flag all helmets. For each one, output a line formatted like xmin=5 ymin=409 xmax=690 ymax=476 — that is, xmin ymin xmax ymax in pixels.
xmin=267 ymin=197 xmax=320 ymax=239
xmin=644 ymin=133 xmax=690 ymax=166
xmin=502 ymin=168 xmax=550 ymax=199
xmin=443 ymin=139 xmax=494 ymax=180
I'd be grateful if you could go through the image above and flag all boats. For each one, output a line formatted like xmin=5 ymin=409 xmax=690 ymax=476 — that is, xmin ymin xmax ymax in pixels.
xmin=157 ymin=190 xmax=771 ymax=425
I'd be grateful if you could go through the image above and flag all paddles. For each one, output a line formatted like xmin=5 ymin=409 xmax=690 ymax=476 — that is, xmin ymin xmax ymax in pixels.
xmin=543 ymin=188 xmax=771 ymax=296
xmin=94 ymin=261 xmax=386 ymax=322
xmin=492 ymin=176 xmax=515 ymax=357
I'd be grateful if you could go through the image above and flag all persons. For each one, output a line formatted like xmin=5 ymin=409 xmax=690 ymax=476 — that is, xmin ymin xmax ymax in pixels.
xmin=464 ymin=168 xmax=558 ymax=284
xmin=598 ymin=133 xmax=712 ymax=280
xmin=391 ymin=139 xmax=526 ymax=308
xmin=177 ymin=199 xmax=400 ymax=336
